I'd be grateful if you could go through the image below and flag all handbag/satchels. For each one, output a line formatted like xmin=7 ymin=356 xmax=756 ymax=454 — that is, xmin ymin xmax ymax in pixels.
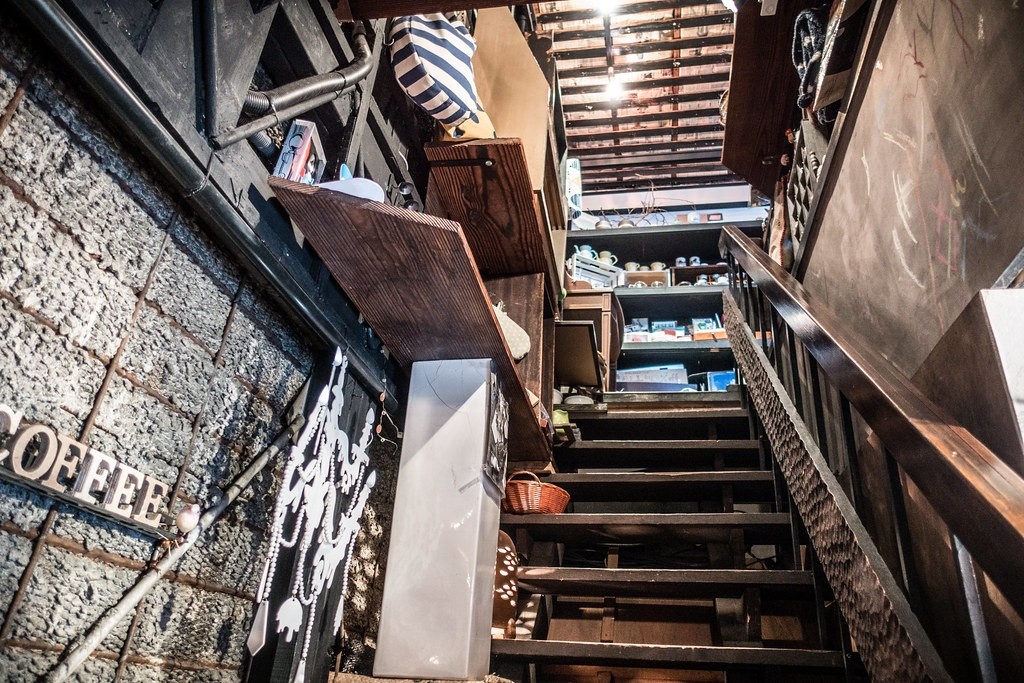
xmin=385 ymin=7 xmax=497 ymax=142
xmin=488 ymin=289 xmax=532 ymax=364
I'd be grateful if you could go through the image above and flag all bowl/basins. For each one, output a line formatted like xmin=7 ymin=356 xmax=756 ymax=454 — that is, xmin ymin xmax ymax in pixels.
xmin=564 ymin=395 xmax=595 ymax=404
xmin=554 ymin=389 xmax=563 ymax=404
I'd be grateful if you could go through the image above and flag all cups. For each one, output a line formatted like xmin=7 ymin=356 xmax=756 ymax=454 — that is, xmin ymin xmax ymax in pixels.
xmin=640 ymin=266 xmax=649 ymax=271
xmin=675 ymin=257 xmax=686 ymax=267
xmin=689 ymin=256 xmax=700 ymax=267
xmin=624 ymin=262 xmax=640 ymax=272
xmin=650 ymin=262 xmax=666 ymax=271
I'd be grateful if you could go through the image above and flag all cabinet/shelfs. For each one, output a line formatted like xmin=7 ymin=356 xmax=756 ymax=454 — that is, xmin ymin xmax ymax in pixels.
xmin=565 ymin=219 xmax=773 ymax=362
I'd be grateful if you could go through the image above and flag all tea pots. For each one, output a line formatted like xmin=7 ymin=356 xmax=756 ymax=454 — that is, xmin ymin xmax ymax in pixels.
xmin=596 ymin=251 xmax=618 ymax=266
xmin=574 ymin=244 xmax=598 ymax=260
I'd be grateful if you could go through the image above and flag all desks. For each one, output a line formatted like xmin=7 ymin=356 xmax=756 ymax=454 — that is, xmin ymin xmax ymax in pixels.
xmin=564 ymin=288 xmax=624 ymax=393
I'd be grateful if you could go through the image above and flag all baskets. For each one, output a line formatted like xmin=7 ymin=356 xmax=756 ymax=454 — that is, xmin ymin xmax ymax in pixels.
xmin=500 ymin=471 xmax=570 ymax=514
